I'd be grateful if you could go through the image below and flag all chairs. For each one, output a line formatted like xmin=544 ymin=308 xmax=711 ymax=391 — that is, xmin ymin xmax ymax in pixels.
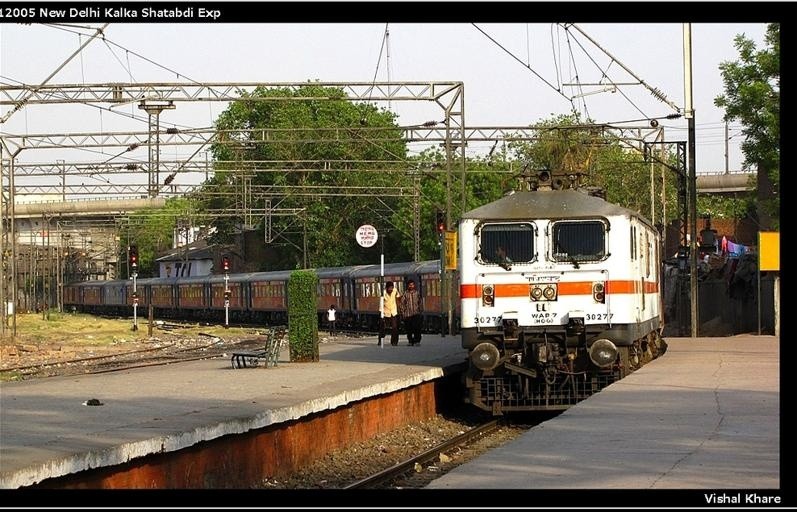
xmin=231 ymin=325 xmax=286 ymax=371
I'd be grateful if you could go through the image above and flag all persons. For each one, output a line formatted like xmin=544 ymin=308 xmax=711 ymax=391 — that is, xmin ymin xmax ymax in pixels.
xmin=494 ymin=240 xmax=512 ymax=263
xmin=326 ymin=304 xmax=337 ymax=336
xmin=401 ymin=280 xmax=424 ymax=346
xmin=379 ymin=281 xmax=401 ymax=346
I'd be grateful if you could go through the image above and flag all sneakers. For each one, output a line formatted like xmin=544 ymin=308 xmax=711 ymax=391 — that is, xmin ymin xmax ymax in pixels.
xmin=407 ymin=343 xmax=420 ymax=346
xmin=392 ymin=342 xmax=397 ymax=346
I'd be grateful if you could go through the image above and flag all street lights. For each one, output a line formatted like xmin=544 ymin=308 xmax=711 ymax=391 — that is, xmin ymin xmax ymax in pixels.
xmin=127 ymin=244 xmax=140 ymax=274
xmin=220 ymin=254 xmax=231 ymax=276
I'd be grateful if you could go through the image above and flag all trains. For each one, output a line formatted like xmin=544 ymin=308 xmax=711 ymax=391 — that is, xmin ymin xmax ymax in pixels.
xmin=63 ymin=168 xmax=665 ymax=415
